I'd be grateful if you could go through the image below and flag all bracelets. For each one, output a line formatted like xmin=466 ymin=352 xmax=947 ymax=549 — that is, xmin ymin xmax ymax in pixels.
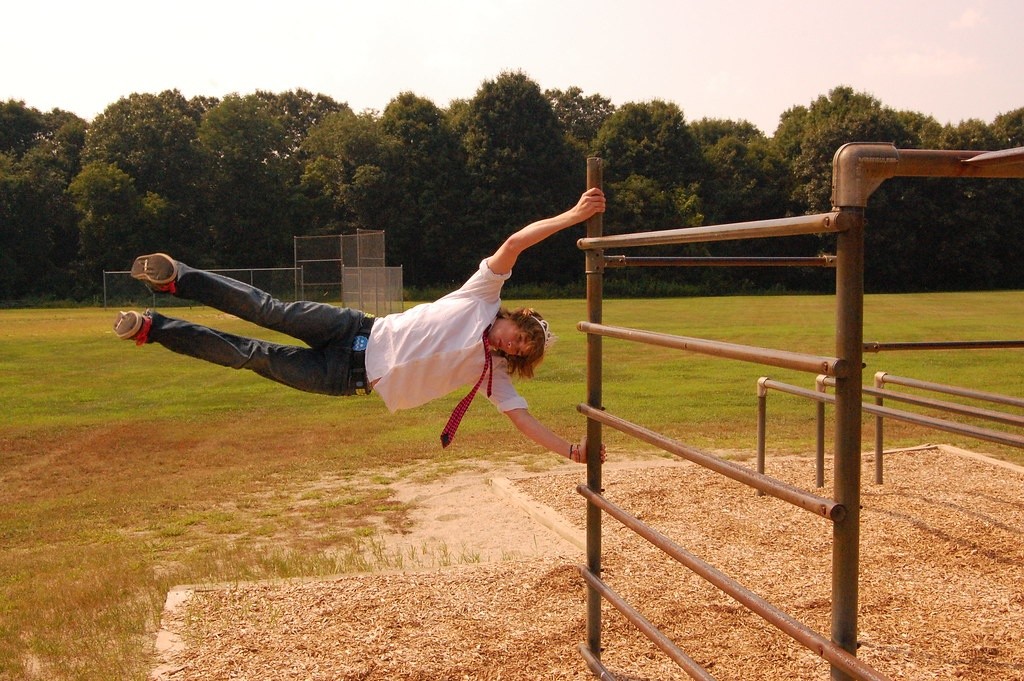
xmin=569 ymin=444 xmax=580 ymax=463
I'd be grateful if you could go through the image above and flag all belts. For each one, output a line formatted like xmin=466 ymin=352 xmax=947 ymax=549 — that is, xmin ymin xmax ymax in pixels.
xmin=351 ymin=313 xmax=374 ymax=396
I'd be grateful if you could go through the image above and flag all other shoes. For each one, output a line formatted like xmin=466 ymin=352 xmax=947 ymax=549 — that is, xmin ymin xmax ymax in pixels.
xmin=113 ymin=310 xmax=151 ymax=347
xmin=131 ymin=253 xmax=178 ymax=294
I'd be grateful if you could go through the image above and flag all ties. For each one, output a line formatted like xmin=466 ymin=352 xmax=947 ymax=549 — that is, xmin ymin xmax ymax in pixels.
xmin=440 ymin=324 xmax=494 ymax=449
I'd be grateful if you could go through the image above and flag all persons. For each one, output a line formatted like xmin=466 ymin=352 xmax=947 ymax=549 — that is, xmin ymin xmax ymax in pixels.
xmin=113 ymin=187 xmax=606 ymax=464
